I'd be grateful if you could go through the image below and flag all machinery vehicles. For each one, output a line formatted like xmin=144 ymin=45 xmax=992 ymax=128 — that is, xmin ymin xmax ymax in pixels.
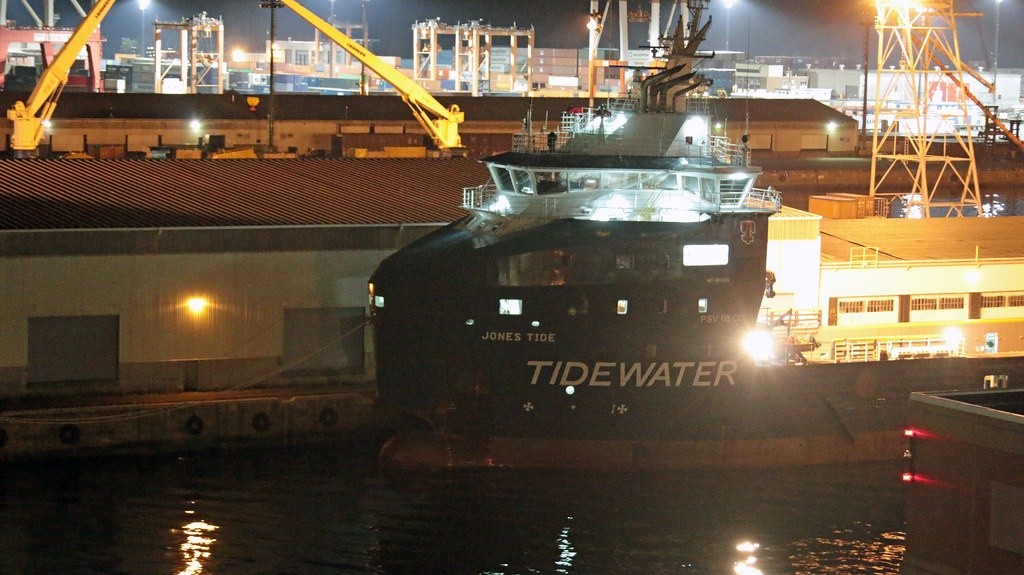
xmin=4 ymin=0 xmax=468 ymax=157
